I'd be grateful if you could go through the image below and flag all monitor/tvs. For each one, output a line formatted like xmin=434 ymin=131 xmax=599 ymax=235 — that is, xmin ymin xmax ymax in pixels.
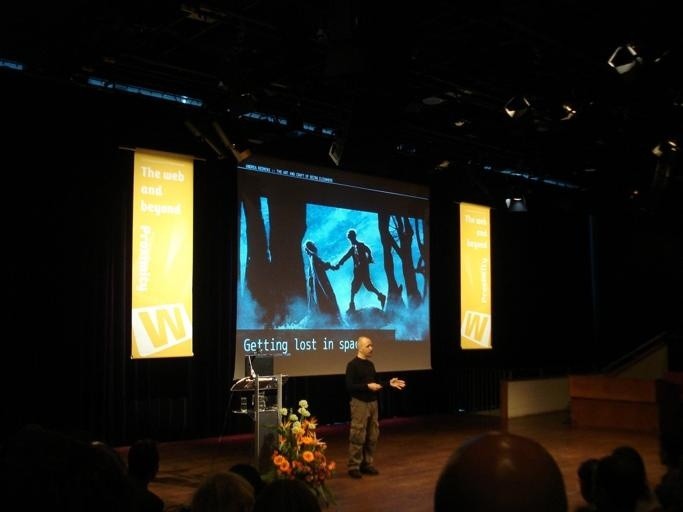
xmin=245 ymin=355 xmax=274 ymax=377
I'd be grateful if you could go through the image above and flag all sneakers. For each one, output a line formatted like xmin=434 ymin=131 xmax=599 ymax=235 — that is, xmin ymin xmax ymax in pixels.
xmin=349 ymin=468 xmax=361 ymax=478
xmin=362 ymin=465 xmax=378 ymax=475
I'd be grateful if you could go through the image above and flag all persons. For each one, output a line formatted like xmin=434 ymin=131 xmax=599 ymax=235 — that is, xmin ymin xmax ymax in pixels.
xmin=345 ymin=336 xmax=406 ymax=481
xmin=2 ymin=421 xmax=321 ymax=512
xmin=338 ymin=228 xmax=385 ymax=313
xmin=305 ymin=240 xmax=342 ymax=324
xmin=432 ymin=432 xmax=682 ymax=511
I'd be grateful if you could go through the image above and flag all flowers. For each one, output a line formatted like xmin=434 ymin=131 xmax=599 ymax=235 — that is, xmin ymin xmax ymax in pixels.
xmin=258 ymin=400 xmax=338 ymax=510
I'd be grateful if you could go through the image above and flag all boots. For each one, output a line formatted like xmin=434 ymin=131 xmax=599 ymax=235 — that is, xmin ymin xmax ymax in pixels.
xmin=378 ymin=293 xmax=386 ymax=309
xmin=346 ymin=302 xmax=356 ymax=313
